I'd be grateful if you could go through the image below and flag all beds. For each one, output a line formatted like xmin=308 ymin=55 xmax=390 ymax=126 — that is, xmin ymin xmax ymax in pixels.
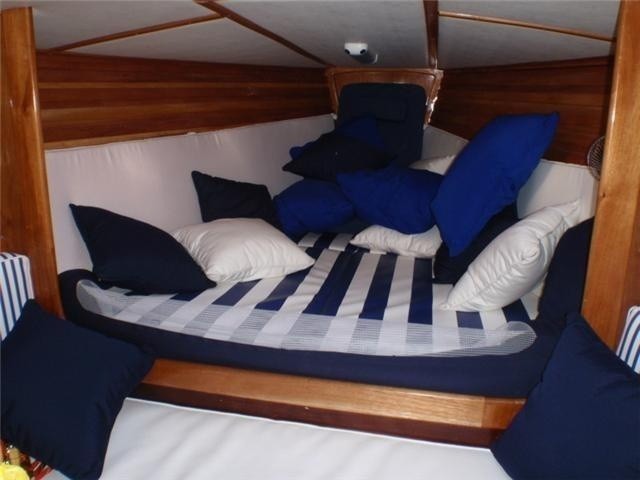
xmin=44 ymin=116 xmax=597 ymax=397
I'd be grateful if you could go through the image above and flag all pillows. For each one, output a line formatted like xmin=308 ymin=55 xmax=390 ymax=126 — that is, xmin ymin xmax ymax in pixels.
xmin=337 ymin=165 xmax=446 ymax=235
xmin=613 ymin=304 xmax=640 ymax=373
xmin=409 ymin=154 xmax=457 ymax=176
xmin=490 ymin=310 xmax=640 ymax=480
xmin=349 ymin=223 xmax=443 ymax=258
xmin=291 ymin=140 xmax=316 ymax=158
xmin=338 ymin=81 xmax=426 ymax=161
xmin=283 ymin=129 xmax=396 ymax=187
xmin=170 ymin=216 xmax=316 ymax=284
xmin=0 ymin=250 xmax=37 ymax=341
xmin=424 ymin=212 xmax=531 ymax=284
xmin=537 ymin=216 xmax=595 ymax=319
xmin=69 ymin=199 xmax=218 ymax=293
xmin=448 ymin=201 xmax=566 ymax=312
xmin=272 ymin=177 xmax=346 ymax=234
xmin=334 ymin=111 xmax=416 ymax=166
xmin=189 ymin=170 xmax=275 ymax=224
xmin=0 ymin=299 xmax=161 ymax=480
xmin=424 ymin=109 xmax=567 ymax=258
